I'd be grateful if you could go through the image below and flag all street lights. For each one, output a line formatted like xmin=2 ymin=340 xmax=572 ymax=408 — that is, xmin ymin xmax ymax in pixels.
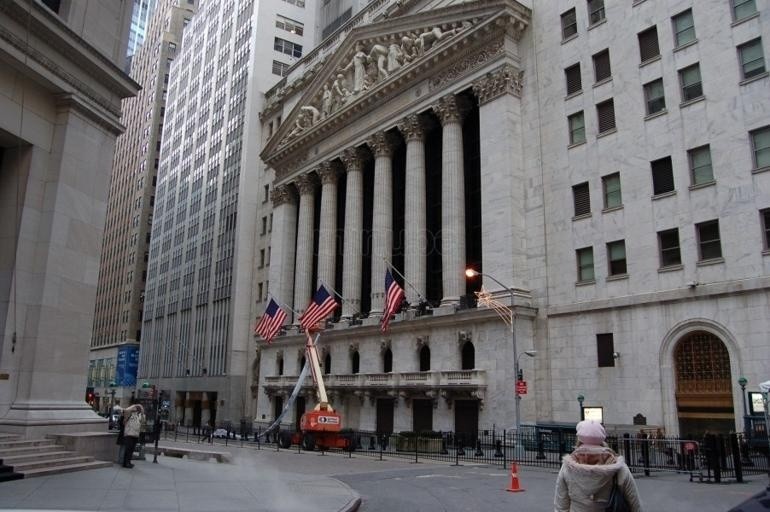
xmin=142 ymin=383 xmax=155 ymax=396
xmin=465 ymin=268 xmax=538 ymax=459
xmin=108 ymin=381 xmax=117 ymax=414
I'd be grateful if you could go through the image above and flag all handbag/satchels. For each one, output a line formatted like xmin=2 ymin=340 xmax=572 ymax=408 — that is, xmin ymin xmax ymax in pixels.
xmin=605 ymin=469 xmax=633 ymax=512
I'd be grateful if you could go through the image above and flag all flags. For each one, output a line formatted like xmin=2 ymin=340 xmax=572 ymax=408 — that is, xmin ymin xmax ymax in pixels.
xmin=253 ymin=298 xmax=288 ymax=348
xmin=294 ymin=284 xmax=338 ymax=334
xmin=379 ymin=269 xmax=406 ymax=335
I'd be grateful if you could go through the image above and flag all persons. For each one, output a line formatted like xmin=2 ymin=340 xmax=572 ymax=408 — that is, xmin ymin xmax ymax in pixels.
xmin=279 ymin=23 xmax=467 ymax=146
xmin=624 ymin=425 xmax=755 ymax=484
xmin=121 ymin=401 xmax=148 ymax=468
xmin=552 ymin=418 xmax=645 ymax=511
xmin=199 ymin=423 xmax=213 ymax=443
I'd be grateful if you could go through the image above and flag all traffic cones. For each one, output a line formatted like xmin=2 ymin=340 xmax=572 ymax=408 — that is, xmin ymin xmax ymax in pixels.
xmin=506 ymin=462 xmax=526 ymax=492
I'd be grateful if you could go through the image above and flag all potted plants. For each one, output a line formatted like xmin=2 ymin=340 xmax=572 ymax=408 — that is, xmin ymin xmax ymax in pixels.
xmin=396 ymin=430 xmax=421 ymax=451
xmin=417 ymin=431 xmax=444 ymax=453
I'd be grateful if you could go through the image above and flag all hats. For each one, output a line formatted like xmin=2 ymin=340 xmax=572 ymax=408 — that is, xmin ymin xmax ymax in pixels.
xmin=575 ymin=420 xmax=607 ymax=444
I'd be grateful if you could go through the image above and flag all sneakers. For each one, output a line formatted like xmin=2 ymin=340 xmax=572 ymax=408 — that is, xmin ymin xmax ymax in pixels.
xmin=121 ymin=463 xmax=135 ymax=469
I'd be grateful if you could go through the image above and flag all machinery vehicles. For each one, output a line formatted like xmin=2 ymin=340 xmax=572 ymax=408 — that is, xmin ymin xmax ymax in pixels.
xmin=279 ymin=328 xmax=356 ymax=451
